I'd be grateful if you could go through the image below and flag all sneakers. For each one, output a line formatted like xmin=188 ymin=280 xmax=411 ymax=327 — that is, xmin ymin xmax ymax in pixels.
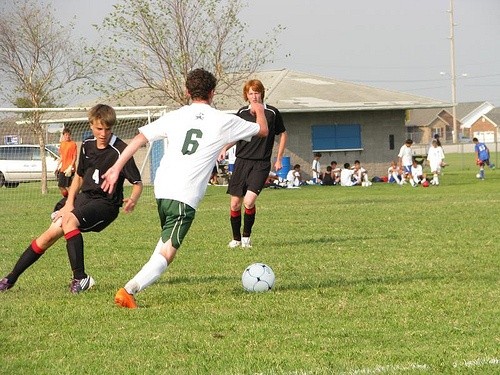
xmin=71 ymin=274 xmax=96 ymax=293
xmin=241 ymin=236 xmax=253 ymax=248
xmin=0 ymin=277 xmax=14 ymax=291
xmin=228 ymin=240 xmax=241 ymax=248
xmin=114 ymin=288 xmax=137 ymax=308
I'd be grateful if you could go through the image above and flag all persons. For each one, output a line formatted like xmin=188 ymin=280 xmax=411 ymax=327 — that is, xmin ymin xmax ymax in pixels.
xmin=312 ymin=152 xmax=321 ymax=185
xmin=266 ymin=171 xmax=288 ymax=187
xmin=0 ymin=104 xmax=143 ymax=291
xmin=226 ymin=144 xmax=236 ymax=173
xmin=410 ymin=160 xmax=430 ymax=184
xmin=473 ymin=137 xmax=495 ymax=178
xmin=288 ymin=164 xmax=305 ymax=187
xmin=217 ymin=79 xmax=287 ymax=249
xmin=208 ymin=164 xmax=218 ymax=184
xmin=341 ymin=159 xmax=373 ymax=187
xmin=100 ymin=68 xmax=268 ymax=308
xmin=388 ymin=161 xmax=407 ymax=184
xmin=54 ymin=129 xmax=77 ymax=198
xmin=398 ymin=139 xmax=418 ymax=187
xmin=427 ymin=134 xmax=446 ymax=186
xmin=322 ymin=161 xmax=342 ymax=186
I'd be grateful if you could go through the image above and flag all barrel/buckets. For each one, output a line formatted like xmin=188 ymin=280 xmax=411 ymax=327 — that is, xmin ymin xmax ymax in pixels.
xmin=275 ymin=156 xmax=290 ymax=177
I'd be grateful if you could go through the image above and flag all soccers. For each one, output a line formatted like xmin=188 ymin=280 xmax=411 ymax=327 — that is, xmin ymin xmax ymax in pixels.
xmin=476 ymin=174 xmax=481 ymax=179
xmin=243 ymin=263 xmax=275 ymax=293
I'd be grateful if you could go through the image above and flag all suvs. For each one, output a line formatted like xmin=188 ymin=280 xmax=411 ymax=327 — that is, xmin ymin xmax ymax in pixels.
xmin=0 ymin=145 xmax=63 ymax=188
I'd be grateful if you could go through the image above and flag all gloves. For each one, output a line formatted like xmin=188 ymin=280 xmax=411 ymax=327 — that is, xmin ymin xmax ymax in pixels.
xmin=54 ymin=167 xmax=59 ymax=175
xmin=64 ymin=165 xmax=72 ymax=177
